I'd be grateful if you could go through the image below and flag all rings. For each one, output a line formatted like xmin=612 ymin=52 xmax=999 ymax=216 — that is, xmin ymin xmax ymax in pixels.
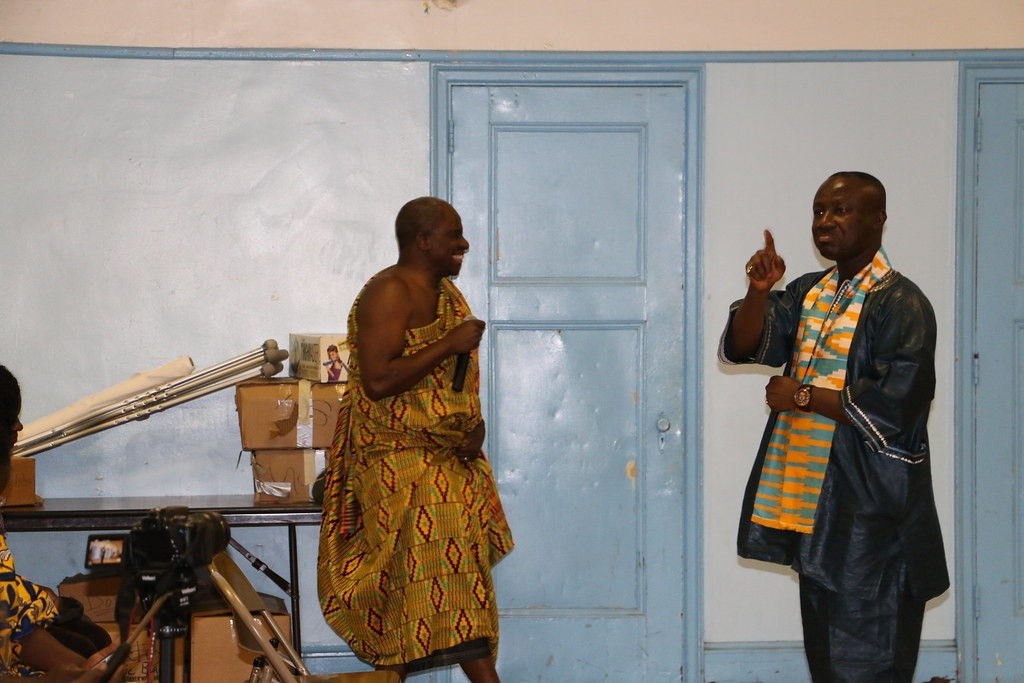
xmin=744 ymin=265 xmax=755 ymax=275
xmin=764 ymin=399 xmax=770 ymax=406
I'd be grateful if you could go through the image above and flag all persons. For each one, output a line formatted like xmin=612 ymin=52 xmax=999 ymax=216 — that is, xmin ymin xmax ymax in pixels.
xmin=325 ymin=346 xmax=342 ymax=382
xmin=87 ymin=542 xmax=122 ymax=563
xmin=317 ymin=197 xmax=516 ymax=683
xmin=716 ymin=172 xmax=950 ymax=682
xmin=0 ymin=365 xmax=128 ymax=683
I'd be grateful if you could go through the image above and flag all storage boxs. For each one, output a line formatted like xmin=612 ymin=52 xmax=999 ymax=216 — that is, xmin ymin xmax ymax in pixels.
xmin=231 ymin=323 xmax=363 ymax=524
xmin=48 ymin=548 xmax=292 ymax=682
xmin=0 ymin=455 xmax=36 ymax=507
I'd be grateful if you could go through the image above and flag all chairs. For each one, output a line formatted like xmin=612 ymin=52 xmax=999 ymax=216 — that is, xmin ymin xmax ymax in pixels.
xmin=206 ymin=547 xmax=404 ymax=683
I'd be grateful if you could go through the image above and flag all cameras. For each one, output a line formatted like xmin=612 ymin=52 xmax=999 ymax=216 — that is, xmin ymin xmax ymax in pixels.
xmin=84 ymin=505 xmax=231 ymax=637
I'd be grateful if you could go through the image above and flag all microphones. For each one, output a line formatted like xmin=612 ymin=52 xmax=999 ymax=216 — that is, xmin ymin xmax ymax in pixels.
xmin=450 ymin=314 xmax=478 ymax=393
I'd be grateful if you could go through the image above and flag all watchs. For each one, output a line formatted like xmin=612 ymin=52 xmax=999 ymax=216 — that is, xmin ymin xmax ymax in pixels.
xmin=793 ymin=385 xmax=815 ymax=412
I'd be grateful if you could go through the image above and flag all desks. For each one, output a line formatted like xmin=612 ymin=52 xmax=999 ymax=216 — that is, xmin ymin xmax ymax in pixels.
xmin=0 ymin=494 xmax=344 ymax=682
